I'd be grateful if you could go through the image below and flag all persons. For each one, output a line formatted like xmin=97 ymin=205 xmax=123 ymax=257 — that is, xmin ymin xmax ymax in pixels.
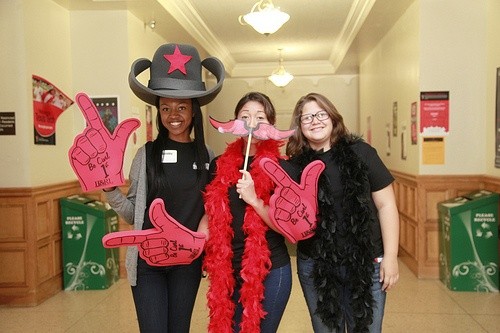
xmin=101 ymin=92 xmax=297 ymax=333
xmin=67 ymin=43 xmax=225 ymax=333
xmin=259 ymin=93 xmax=400 ymax=333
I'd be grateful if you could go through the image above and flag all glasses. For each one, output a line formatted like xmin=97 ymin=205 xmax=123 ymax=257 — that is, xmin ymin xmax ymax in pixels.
xmin=298 ymin=110 xmax=330 ymax=125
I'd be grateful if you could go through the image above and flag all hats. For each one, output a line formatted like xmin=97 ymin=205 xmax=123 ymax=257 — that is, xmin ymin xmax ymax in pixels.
xmin=128 ymin=43 xmax=226 ymax=107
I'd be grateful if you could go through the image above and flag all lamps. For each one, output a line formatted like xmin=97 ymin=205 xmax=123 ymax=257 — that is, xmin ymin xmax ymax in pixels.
xmin=268 ymin=48 xmax=294 ymax=88
xmin=238 ymin=0 xmax=290 ymax=36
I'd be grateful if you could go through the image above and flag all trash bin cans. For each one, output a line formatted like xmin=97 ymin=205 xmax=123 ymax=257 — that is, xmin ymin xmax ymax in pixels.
xmin=59 ymin=193 xmax=120 ymax=291
xmin=438 ymin=187 xmax=500 ymax=292
